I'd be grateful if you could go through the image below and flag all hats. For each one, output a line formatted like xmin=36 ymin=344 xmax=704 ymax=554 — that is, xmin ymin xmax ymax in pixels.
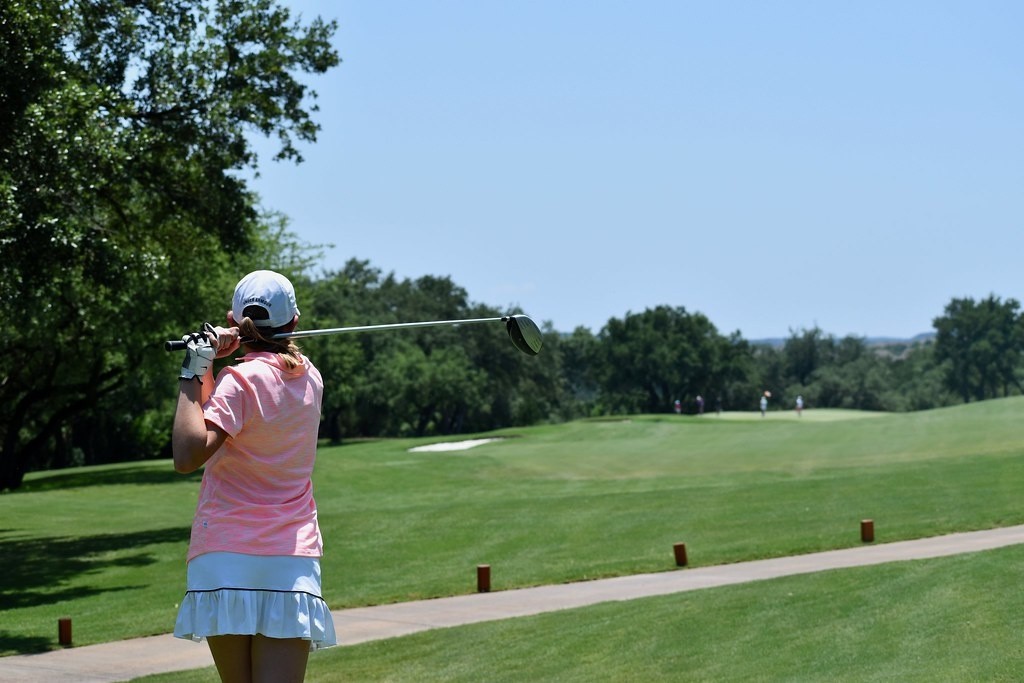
xmin=232 ymin=269 xmax=300 ymax=327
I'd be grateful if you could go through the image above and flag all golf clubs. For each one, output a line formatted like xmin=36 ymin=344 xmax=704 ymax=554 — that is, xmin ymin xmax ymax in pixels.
xmin=164 ymin=314 xmax=545 ymax=352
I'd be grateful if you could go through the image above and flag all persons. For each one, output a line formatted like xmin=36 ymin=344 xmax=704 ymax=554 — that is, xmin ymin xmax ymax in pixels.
xmin=172 ymin=271 xmax=337 ymax=683
xmin=696 ymin=396 xmax=703 ymax=414
xmin=716 ymin=396 xmax=721 ymax=413
xmin=796 ymin=396 xmax=803 ymax=418
xmin=760 ymin=396 xmax=767 ymax=417
xmin=675 ymin=400 xmax=681 ymax=414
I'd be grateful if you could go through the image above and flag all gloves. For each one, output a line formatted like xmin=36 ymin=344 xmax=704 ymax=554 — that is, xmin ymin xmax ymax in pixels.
xmin=179 ymin=321 xmax=220 ymax=383
